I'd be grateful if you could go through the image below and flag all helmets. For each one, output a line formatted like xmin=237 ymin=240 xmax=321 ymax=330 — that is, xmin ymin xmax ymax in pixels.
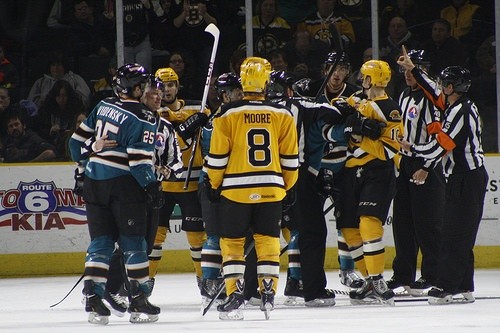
xmin=240 ymin=57 xmax=271 ymax=92
xmin=439 ymin=66 xmax=473 ymax=94
xmin=155 ymin=68 xmax=180 ymax=88
xmin=322 ymin=49 xmax=351 ymax=69
xmin=265 ymin=70 xmax=320 ymax=103
xmin=214 ymin=71 xmax=241 ymax=103
xmin=145 ymin=73 xmax=165 ymax=91
xmin=407 ymin=49 xmax=431 ymax=65
xmin=359 ymin=60 xmax=391 ymax=87
xmin=112 ymin=63 xmax=151 ymax=94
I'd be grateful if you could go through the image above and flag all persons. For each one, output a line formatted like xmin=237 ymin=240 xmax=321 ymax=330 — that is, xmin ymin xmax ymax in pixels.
xmin=398 ymin=45 xmax=488 ymax=305
xmin=68 ymin=63 xmax=160 ymax=324
xmin=199 ymin=71 xmax=263 ymax=305
xmin=384 ymin=51 xmax=442 ymax=297
xmin=268 ymin=70 xmax=387 ymax=308
xmin=148 ymin=68 xmax=211 ymax=295
xmin=305 ymin=49 xmax=366 ymax=289
xmin=1 ymin=0 xmax=497 ymax=162
xmin=207 ymin=56 xmax=299 ymax=321
xmin=80 ymin=75 xmax=183 ymax=313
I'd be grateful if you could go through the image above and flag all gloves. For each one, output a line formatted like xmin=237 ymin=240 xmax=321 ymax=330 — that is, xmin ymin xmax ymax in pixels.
xmin=335 ymin=100 xmax=361 ymax=122
xmin=175 ymin=112 xmax=209 ymax=137
xmin=73 ymin=168 xmax=85 ymax=196
xmin=281 ymin=187 xmax=296 ymax=207
xmin=352 ymin=111 xmax=383 ymax=140
xmin=203 ymin=174 xmax=223 ymax=203
xmin=316 ymin=168 xmax=334 ymax=198
xmin=144 ymin=181 xmax=165 ymax=209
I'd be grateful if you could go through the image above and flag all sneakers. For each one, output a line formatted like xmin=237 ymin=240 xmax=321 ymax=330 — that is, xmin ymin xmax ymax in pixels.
xmin=197 ymin=272 xmax=475 ymax=310
xmin=104 ymin=283 xmax=127 ymax=317
xmin=128 ymin=282 xmax=161 ymax=324
xmin=81 ymin=282 xmax=111 ymax=324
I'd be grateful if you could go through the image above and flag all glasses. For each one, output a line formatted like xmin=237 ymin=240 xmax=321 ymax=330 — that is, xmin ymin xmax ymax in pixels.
xmin=168 ymin=59 xmax=184 ymax=64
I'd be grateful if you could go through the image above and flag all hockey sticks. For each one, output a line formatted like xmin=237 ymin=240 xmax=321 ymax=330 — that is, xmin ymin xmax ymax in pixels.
xmin=183 ymin=23 xmax=220 ymax=190
xmin=201 ymin=239 xmax=256 ymax=315
xmin=280 ymin=201 xmax=335 ymax=256
xmin=314 ymin=23 xmax=344 ymax=101
xmin=49 ymin=272 xmax=86 ymax=307
